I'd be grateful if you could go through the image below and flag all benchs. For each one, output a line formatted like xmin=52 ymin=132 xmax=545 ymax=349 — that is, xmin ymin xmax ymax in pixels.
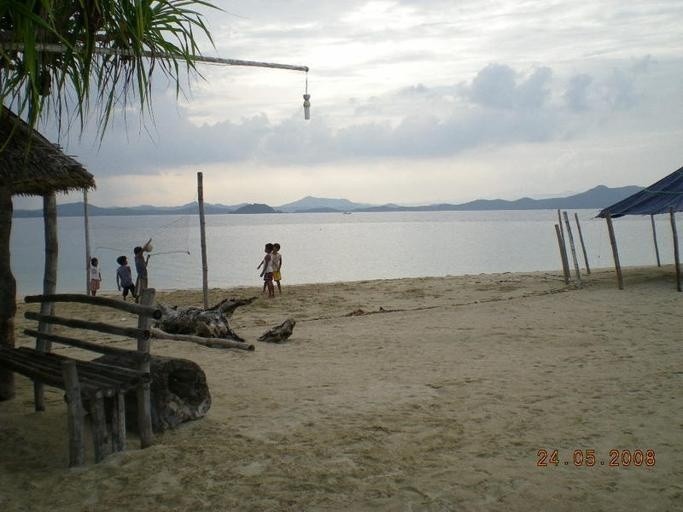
xmin=1 ymin=293 xmax=163 ymax=467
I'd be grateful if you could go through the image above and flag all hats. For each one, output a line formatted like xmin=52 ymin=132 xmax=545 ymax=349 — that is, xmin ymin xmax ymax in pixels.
xmin=141 ymin=238 xmax=154 ymax=253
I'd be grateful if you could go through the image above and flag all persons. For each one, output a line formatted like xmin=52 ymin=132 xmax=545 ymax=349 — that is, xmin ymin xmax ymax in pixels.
xmin=260 ymin=242 xmax=276 ymax=298
xmin=132 ymin=238 xmax=151 ymax=303
xmin=115 ymin=256 xmax=134 ymax=301
xmin=255 ymin=242 xmax=283 ymax=295
xmin=87 ymin=255 xmax=101 ymax=297
